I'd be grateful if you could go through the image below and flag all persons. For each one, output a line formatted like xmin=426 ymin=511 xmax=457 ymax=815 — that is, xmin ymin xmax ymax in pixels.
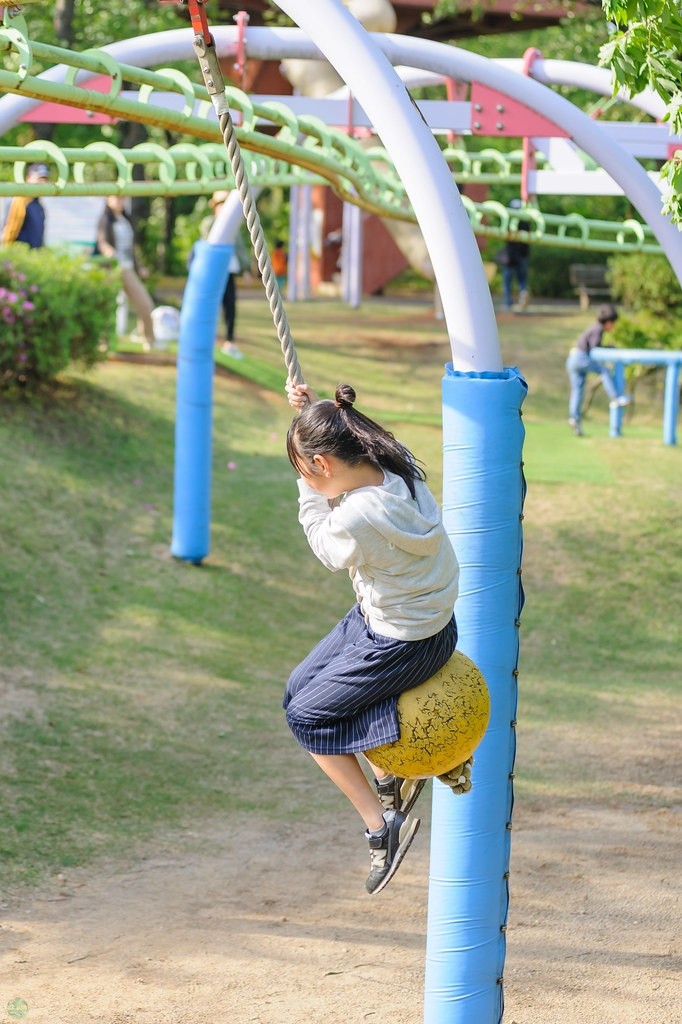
xmin=0 ymin=164 xmax=48 ymax=250
xmin=563 ymin=303 xmax=631 ymax=436
xmin=187 ymin=189 xmax=243 ymax=360
xmin=270 ymin=240 xmax=289 ymax=299
xmin=498 ymin=198 xmax=531 ymax=314
xmin=89 ymin=194 xmax=167 ymax=355
xmin=286 ymin=374 xmax=461 ymax=895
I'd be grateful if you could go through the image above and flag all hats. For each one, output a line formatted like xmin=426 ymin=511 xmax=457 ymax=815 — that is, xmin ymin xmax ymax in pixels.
xmin=207 ymin=190 xmax=228 ymax=209
xmin=27 ymin=162 xmax=49 ymax=178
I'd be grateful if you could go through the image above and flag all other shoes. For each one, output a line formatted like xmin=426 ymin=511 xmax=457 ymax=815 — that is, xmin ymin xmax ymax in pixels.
xmin=142 ymin=342 xmax=169 ymax=352
xmin=220 ymin=342 xmax=246 ymax=359
xmin=609 ymin=394 xmax=632 ymax=409
xmin=568 ymin=416 xmax=582 ymax=436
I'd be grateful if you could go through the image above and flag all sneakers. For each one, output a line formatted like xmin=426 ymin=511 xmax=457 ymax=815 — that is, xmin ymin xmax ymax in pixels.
xmin=373 ymin=775 xmax=428 ymax=815
xmin=364 ymin=807 xmax=420 ymax=896
